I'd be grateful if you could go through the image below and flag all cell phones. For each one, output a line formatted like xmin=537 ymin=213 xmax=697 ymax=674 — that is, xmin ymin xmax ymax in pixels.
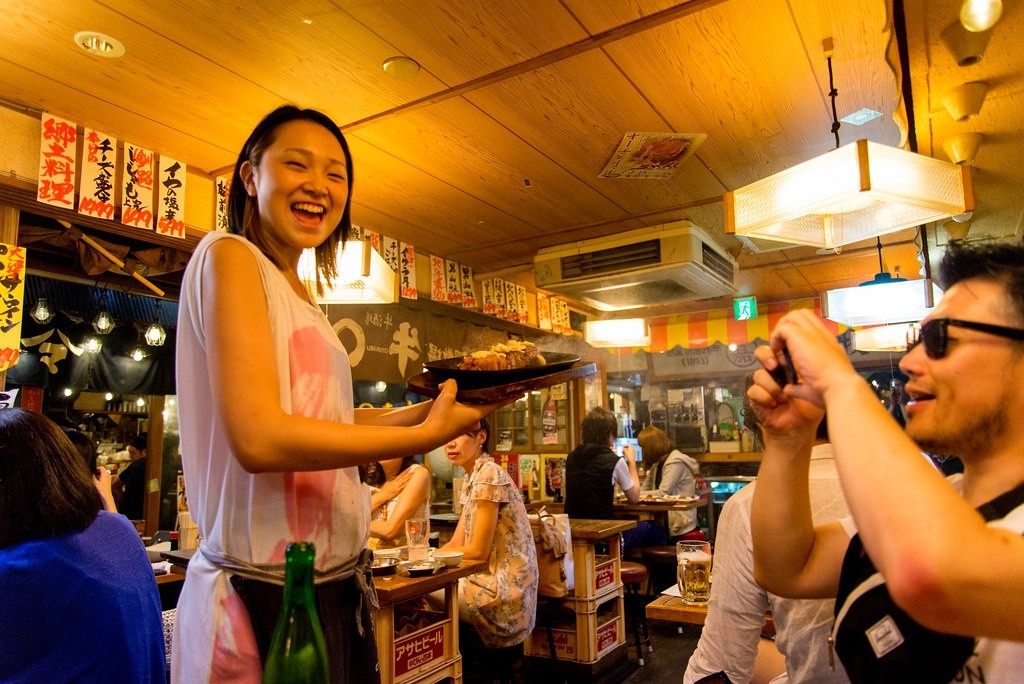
xmin=767 ymin=348 xmax=798 ymax=388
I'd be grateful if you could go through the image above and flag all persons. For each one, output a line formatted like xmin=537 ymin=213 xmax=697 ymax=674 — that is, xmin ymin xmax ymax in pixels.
xmin=403 ymin=415 xmax=537 ymax=650
xmin=0 ymin=407 xmax=182 ymax=684
xmin=682 ymin=238 xmax=1024 ymax=684
xmin=361 ymin=454 xmax=431 ymax=550
xmin=564 ymin=408 xmax=661 ymax=569
xmin=638 ymin=425 xmax=700 ymax=546
xmin=169 ymin=104 xmax=527 ymax=684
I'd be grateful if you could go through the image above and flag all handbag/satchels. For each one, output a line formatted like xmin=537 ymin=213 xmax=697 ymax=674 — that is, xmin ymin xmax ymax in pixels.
xmin=527 ymin=506 xmax=575 ymax=597
xmin=826 ymin=532 xmax=977 ymax=684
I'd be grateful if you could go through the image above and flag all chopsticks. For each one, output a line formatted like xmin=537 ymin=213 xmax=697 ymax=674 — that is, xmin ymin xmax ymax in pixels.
xmin=179 ymin=511 xmax=197 ymax=527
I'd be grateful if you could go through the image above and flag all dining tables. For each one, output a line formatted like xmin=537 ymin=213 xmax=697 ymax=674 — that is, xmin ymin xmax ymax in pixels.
xmin=645 ymin=581 xmax=780 ymax=635
xmin=427 ymin=517 xmax=635 ymax=676
xmin=171 ymin=558 xmax=487 ymax=684
xmin=537 ymin=494 xmax=708 ymax=551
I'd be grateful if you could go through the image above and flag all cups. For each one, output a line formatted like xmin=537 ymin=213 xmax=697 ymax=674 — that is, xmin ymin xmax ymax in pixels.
xmin=676 ymin=539 xmax=712 ymax=607
xmin=178 ymin=527 xmax=197 ymax=550
xmin=405 ymin=517 xmax=430 ymax=560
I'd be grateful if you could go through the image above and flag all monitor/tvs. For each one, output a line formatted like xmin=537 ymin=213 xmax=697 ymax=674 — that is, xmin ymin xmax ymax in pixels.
xmin=612 ymin=437 xmax=643 ymax=462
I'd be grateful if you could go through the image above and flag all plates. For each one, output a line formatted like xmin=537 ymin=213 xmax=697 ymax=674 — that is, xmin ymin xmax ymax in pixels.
xmin=616 ymin=497 xmax=699 ymax=505
xmin=404 ymin=562 xmax=446 ymax=576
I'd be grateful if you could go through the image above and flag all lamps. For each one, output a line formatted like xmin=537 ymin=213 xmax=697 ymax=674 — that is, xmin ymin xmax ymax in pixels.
xmin=724 ymin=55 xmax=974 ymax=248
xmin=819 ymin=233 xmax=945 ymax=354
xmin=27 ymin=282 xmax=167 ymax=346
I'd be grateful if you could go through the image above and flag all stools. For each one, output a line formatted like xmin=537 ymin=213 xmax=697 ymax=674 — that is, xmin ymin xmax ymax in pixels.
xmin=642 ymin=545 xmax=683 ymax=634
xmin=620 ymin=559 xmax=651 ymax=666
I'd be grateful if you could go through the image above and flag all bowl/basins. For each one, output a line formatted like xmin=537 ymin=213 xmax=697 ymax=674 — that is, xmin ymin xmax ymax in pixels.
xmin=371 ymin=557 xmax=400 ymax=574
xmin=422 ymin=352 xmax=581 ymax=387
xmin=373 ymin=548 xmax=401 ymax=558
xmin=433 ymin=551 xmax=464 ymax=568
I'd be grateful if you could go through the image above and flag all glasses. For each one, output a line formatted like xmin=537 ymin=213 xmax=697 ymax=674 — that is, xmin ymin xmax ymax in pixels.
xmin=905 ymin=317 xmax=1023 ymax=360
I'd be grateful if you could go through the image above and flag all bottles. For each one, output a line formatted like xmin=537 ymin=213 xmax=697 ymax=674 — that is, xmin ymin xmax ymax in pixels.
xmin=170 ymin=531 xmax=179 ymax=550
xmin=711 ymin=424 xmax=719 ymax=442
xmin=741 ymin=430 xmax=751 ymax=452
xmin=522 ymin=484 xmax=529 ymax=502
xmin=730 ymin=421 xmax=740 ymax=441
xmin=262 ymin=541 xmax=330 ymax=684
xmin=553 ymin=482 xmax=562 ymax=502
